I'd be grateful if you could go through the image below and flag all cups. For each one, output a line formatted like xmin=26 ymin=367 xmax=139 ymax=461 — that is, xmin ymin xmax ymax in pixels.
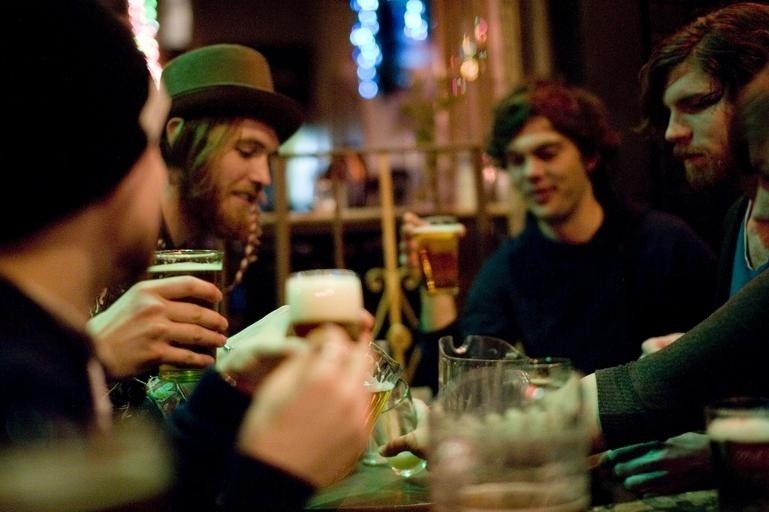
xmin=367 ymin=335 xmax=593 ymax=510
xmin=146 ymin=248 xmax=225 ymax=384
xmin=285 ymin=267 xmax=363 ymax=343
xmin=417 ymin=215 xmax=462 ymax=299
xmin=706 ymin=396 xmax=768 ymax=512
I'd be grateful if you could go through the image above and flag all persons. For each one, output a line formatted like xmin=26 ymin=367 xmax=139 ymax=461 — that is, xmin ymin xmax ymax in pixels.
xmin=0 ymin=1 xmax=376 ymax=512
xmin=378 ymin=58 xmax=769 ymax=489
xmin=86 ymin=43 xmax=304 ymax=443
xmin=397 ymin=78 xmax=716 ymax=452
xmin=635 ymin=3 xmax=769 ymax=358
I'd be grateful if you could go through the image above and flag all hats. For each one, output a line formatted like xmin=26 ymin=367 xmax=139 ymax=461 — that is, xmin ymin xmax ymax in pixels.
xmin=161 ymin=43 xmax=305 ymax=145
